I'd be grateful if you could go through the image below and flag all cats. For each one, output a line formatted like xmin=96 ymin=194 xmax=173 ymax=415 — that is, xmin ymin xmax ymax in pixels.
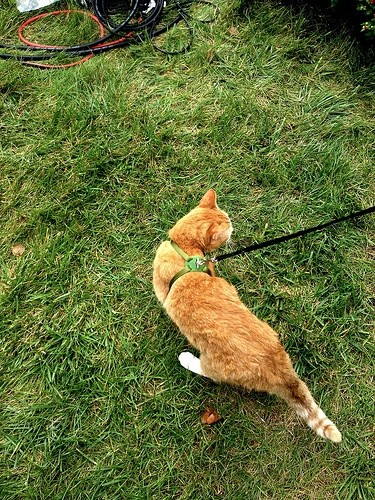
xmin=152 ymin=188 xmax=343 ymax=444
